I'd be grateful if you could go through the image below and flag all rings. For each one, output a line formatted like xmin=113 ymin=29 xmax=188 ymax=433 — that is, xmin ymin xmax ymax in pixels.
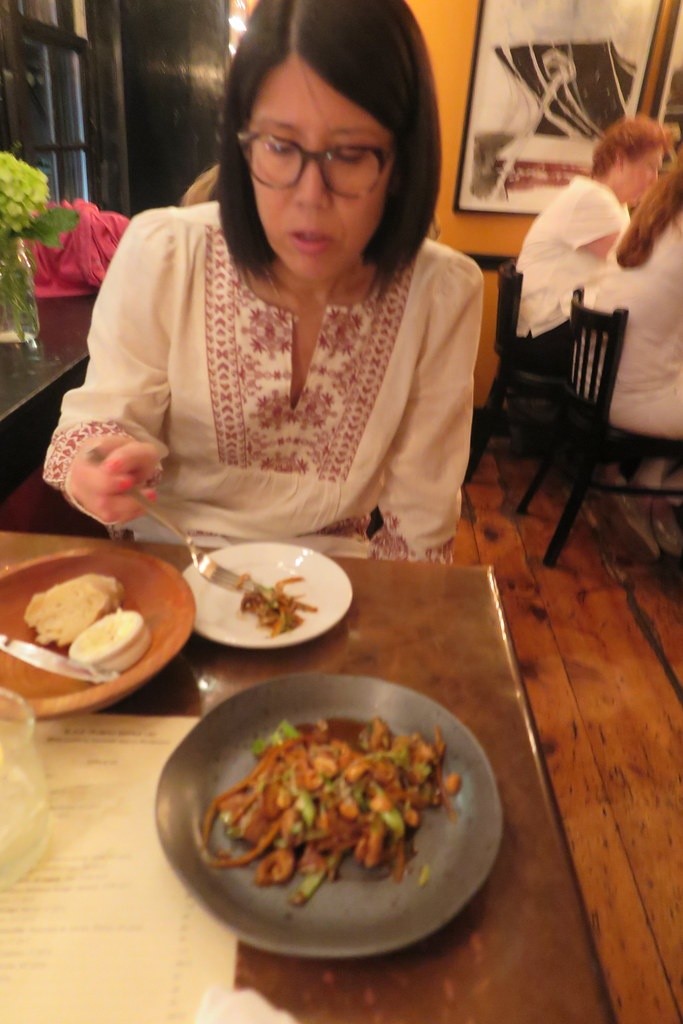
xmin=85 ymin=444 xmax=104 ymax=461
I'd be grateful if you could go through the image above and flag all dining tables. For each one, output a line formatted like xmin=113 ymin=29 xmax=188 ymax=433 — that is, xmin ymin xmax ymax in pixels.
xmin=0 ymin=531 xmax=620 ymax=1024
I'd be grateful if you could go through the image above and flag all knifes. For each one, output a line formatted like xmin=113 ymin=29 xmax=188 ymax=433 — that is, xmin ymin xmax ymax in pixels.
xmin=1 ymin=636 xmax=112 ymax=681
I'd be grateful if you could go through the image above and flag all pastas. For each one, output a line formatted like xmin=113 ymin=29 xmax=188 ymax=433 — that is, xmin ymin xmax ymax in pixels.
xmin=201 ymin=727 xmax=447 ymax=886
xmin=237 ymin=574 xmax=317 ymax=635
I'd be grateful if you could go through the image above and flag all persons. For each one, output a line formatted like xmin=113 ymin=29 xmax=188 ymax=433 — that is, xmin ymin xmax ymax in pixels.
xmin=573 ymin=145 xmax=683 ymax=561
xmin=516 ymin=115 xmax=668 ymax=378
xmin=42 ymin=0 xmax=484 ymax=562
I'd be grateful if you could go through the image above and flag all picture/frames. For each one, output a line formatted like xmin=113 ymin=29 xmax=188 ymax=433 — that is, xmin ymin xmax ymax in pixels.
xmin=453 ymin=0 xmax=664 ymax=215
xmin=650 ymin=0 xmax=683 ymax=163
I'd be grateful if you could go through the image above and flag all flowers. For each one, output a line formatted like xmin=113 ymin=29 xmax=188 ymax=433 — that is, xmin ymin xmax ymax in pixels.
xmin=0 ymin=140 xmax=81 ymax=342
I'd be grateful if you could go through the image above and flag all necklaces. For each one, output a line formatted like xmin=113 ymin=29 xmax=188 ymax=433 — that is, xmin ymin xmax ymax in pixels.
xmin=266 ymin=264 xmax=335 ymax=322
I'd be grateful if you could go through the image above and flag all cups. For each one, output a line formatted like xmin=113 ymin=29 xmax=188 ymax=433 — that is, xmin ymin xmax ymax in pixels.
xmin=0 ymin=691 xmax=57 ymax=887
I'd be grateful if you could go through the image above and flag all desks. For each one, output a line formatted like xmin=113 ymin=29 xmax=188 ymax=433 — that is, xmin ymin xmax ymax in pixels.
xmin=0 ymin=293 xmax=97 ymax=536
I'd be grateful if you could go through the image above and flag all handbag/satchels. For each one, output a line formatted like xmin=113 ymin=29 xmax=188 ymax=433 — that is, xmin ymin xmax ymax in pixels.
xmin=21 ymin=199 xmax=133 ymax=299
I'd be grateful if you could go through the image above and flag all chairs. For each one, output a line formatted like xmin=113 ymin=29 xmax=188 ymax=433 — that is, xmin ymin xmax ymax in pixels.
xmin=466 ymin=254 xmax=566 ymax=491
xmin=515 ymin=286 xmax=683 ymax=572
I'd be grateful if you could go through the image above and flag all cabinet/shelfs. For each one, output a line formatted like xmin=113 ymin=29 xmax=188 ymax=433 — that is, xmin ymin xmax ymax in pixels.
xmin=0 ymin=0 xmax=96 ymax=206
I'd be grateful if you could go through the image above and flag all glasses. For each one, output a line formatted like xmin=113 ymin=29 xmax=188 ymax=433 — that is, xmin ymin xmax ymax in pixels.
xmin=238 ymin=130 xmax=398 ymax=197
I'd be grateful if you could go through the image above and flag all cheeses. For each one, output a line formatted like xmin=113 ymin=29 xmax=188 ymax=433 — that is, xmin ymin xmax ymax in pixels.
xmin=23 ymin=573 xmax=124 ymax=647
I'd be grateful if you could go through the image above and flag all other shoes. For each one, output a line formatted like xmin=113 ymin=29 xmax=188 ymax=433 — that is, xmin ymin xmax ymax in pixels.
xmin=607 ymin=477 xmax=661 ymax=562
xmin=649 ymin=496 xmax=683 ymax=558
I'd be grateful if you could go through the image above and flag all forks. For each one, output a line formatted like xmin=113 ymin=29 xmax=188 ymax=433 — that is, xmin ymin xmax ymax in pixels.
xmin=89 ymin=448 xmax=261 ymax=594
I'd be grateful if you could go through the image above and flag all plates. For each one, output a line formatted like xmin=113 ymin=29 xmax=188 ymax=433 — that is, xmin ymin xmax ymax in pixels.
xmin=182 ymin=543 xmax=352 ymax=648
xmin=154 ymin=672 xmax=505 ymax=958
xmin=1 ymin=546 xmax=197 ymax=720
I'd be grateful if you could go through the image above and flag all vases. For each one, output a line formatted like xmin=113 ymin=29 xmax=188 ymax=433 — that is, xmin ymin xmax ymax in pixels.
xmin=0 ymin=237 xmax=41 ymax=342
